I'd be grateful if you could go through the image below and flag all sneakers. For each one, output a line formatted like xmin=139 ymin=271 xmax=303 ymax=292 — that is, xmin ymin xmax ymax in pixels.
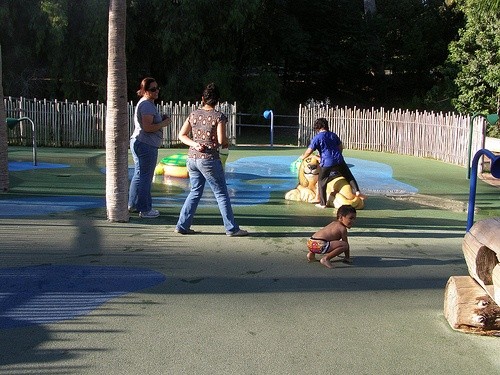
xmin=128 ymin=206 xmax=139 ymax=212
xmin=226 ymin=229 xmax=248 ymax=236
xmin=174 ymin=225 xmax=194 ymax=234
xmin=139 ymin=209 xmax=160 ymax=218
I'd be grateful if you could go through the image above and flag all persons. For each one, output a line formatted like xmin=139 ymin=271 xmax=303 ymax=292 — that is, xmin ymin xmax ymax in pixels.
xmin=306 ymin=205 xmax=356 ymax=269
xmin=175 ymin=84 xmax=249 ymax=237
xmin=299 ymin=118 xmax=367 ymax=208
xmin=127 ymin=78 xmax=172 ymax=218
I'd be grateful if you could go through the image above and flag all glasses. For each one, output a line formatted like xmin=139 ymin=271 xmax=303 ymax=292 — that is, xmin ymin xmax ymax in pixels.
xmin=147 ymin=85 xmax=160 ymax=92
